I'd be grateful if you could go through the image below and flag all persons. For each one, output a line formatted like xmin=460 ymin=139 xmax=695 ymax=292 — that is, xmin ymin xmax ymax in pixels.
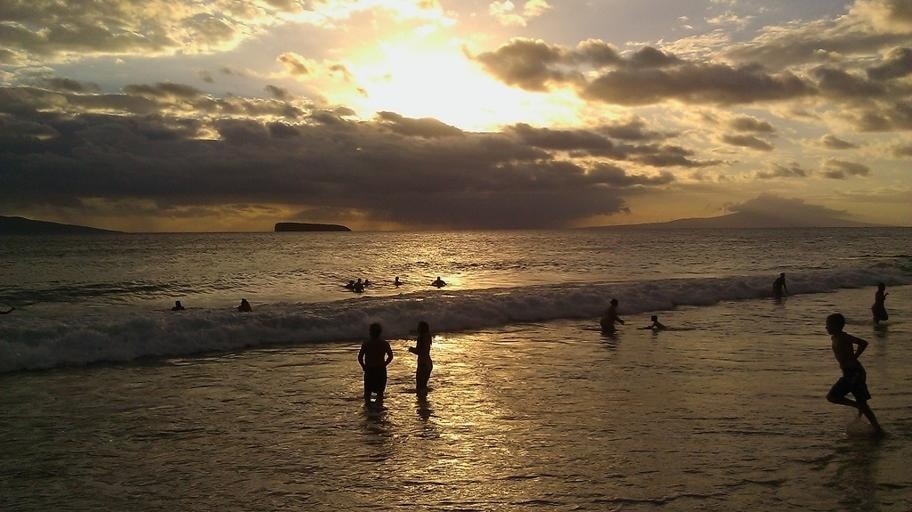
xmin=353 ymin=278 xmax=364 ymax=292
xmin=431 ymin=276 xmax=447 ymax=285
xmin=770 ymin=272 xmax=788 ymax=297
xmin=647 ymin=315 xmax=666 ymax=329
xmin=599 ymin=297 xmax=624 ymax=329
xmin=870 ymin=282 xmax=889 ymax=325
xmin=346 ymin=280 xmax=354 ymax=289
xmin=824 ymin=312 xmax=882 ymax=434
xmin=407 ymin=320 xmax=434 ymax=392
xmin=237 ymin=298 xmax=252 ymax=312
xmin=392 ymin=276 xmax=400 ymax=284
xmin=171 ymin=300 xmax=184 ymax=312
xmin=357 ymin=321 xmax=393 ymax=406
